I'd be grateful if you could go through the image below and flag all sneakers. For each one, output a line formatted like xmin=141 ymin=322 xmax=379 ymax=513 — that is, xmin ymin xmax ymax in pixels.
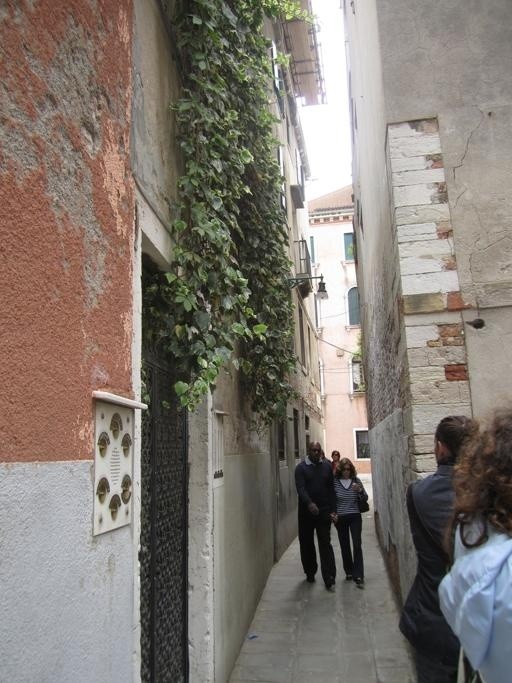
xmin=326 ymin=576 xmax=335 ymax=588
xmin=354 ymin=577 xmax=364 ymax=584
xmin=306 ymin=574 xmax=315 ymax=584
xmin=346 ymin=574 xmax=353 ymax=581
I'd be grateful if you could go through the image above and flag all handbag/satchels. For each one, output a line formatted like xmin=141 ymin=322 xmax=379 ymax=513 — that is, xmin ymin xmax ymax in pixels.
xmin=358 ymin=490 xmax=370 ymax=514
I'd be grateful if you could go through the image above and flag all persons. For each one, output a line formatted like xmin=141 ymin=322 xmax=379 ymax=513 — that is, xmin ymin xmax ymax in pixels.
xmin=437 ymin=402 xmax=511 ymax=683
xmin=397 ymin=415 xmax=481 ymax=683
xmin=331 ymin=451 xmax=341 ymax=475
xmin=330 ymin=458 xmax=368 ymax=586
xmin=321 ymin=449 xmax=331 ymax=463
xmin=294 ymin=441 xmax=338 ymax=589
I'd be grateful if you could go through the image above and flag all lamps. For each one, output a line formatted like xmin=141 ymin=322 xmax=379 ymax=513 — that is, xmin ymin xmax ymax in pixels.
xmin=290 ymin=271 xmax=329 ymax=300
